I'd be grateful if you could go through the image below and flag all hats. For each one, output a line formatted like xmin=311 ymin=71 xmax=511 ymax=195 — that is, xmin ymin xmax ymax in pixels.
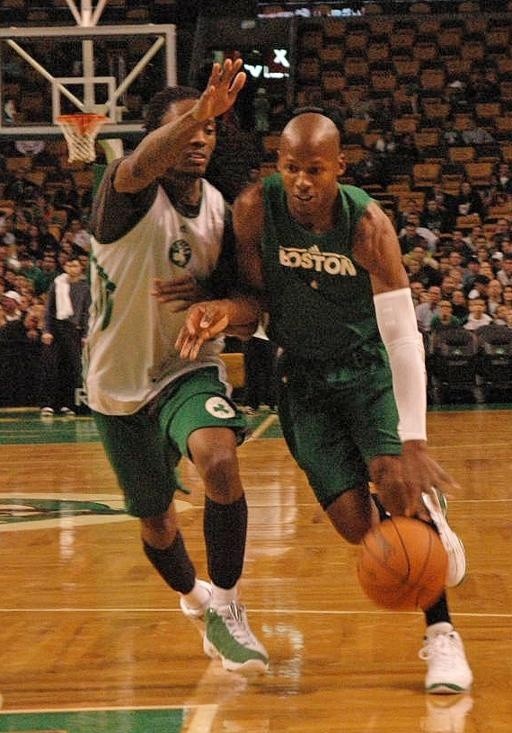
xmin=491 ymin=251 xmax=504 ymax=262
xmin=2 ymin=290 xmax=22 ymax=306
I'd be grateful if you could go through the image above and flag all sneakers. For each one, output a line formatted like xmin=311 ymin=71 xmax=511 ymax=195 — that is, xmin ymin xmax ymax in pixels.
xmin=202 ymin=603 xmax=270 ymax=673
xmin=423 ymin=485 xmax=468 ymax=590
xmin=180 ymin=581 xmax=216 ymax=658
xmin=423 ymin=621 xmax=474 ymax=693
xmin=41 ymin=408 xmax=54 ymax=416
xmin=61 ymin=407 xmax=75 ymax=416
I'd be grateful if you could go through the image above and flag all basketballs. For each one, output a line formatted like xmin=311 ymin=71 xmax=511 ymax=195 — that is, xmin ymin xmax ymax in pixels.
xmin=357 ymin=516 xmax=448 ymax=612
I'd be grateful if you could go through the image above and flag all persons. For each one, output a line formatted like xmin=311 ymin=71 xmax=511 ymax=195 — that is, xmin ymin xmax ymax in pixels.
xmin=178 ymin=655 xmax=275 ymax=731
xmin=330 ymin=103 xmax=511 ymax=402
xmin=218 ymin=104 xmax=291 ymax=188
xmin=423 ymin=692 xmax=474 ymax=733
xmin=236 ymin=313 xmax=279 ymax=416
xmin=0 ymin=140 xmax=107 ymax=421
xmin=173 ymin=102 xmax=476 ymax=695
xmin=85 ymin=57 xmax=272 ymax=679
